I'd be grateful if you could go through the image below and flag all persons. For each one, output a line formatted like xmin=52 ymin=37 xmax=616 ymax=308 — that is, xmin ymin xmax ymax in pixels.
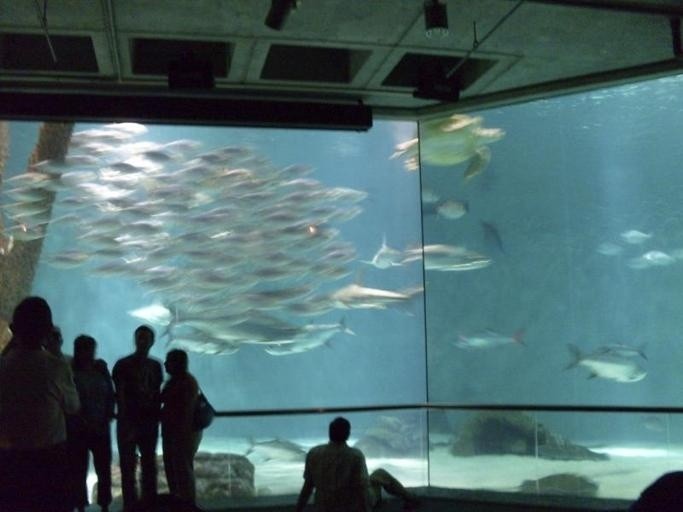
xmin=59 ymin=335 xmax=115 ymax=512
xmin=41 ymin=321 xmax=68 ymax=361
xmin=0 ymin=296 xmax=80 ymax=512
xmin=111 ymin=325 xmax=163 ymax=512
xmin=159 ymin=348 xmax=203 ymax=512
xmin=296 ymin=416 xmax=423 ymax=512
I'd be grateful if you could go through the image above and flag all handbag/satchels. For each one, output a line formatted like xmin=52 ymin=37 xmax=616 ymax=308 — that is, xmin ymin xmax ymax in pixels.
xmin=173 ymin=388 xmax=216 ymax=433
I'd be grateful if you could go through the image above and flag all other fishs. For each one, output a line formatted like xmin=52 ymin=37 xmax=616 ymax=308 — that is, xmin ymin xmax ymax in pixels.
xmin=0 ymin=118 xmax=372 ymax=358
